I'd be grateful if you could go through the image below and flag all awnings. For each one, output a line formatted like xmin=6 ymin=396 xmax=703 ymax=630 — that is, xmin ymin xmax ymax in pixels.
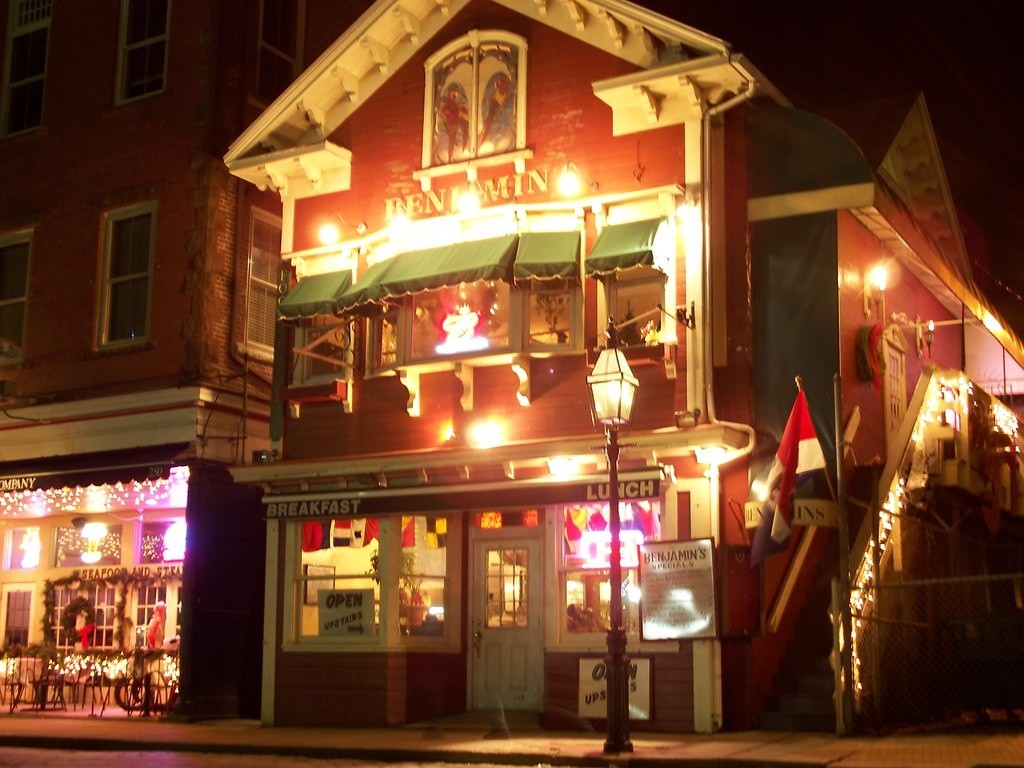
xmin=276 ymin=215 xmax=670 ymax=326
xmin=0 ymin=440 xmax=193 ymax=496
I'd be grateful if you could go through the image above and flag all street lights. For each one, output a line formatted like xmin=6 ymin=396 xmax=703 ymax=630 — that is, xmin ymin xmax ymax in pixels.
xmin=586 ymin=314 xmax=658 ymax=756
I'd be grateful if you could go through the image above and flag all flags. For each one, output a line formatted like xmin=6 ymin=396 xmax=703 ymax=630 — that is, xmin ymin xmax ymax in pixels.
xmin=748 ymin=390 xmax=841 ymax=574
